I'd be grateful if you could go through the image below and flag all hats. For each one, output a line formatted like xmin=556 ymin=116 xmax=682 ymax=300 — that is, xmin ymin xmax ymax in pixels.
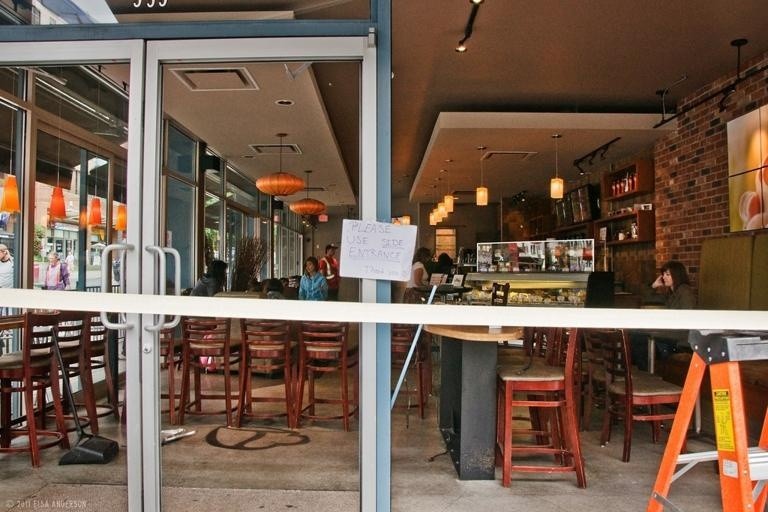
xmin=326 ymin=244 xmax=339 ymax=249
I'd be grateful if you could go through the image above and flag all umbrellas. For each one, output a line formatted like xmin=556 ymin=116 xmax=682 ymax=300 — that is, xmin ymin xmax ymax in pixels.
xmin=90 ymin=243 xmax=106 ymax=255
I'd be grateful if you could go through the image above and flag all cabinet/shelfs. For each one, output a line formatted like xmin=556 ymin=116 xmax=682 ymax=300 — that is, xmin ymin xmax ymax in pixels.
xmin=596 ymin=157 xmax=655 ymax=245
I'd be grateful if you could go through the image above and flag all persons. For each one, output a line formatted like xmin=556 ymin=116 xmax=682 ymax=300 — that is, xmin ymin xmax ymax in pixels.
xmin=402 ymin=247 xmax=431 ymax=304
xmin=44 ymin=251 xmax=71 ymax=291
xmin=0 ymin=243 xmax=14 ymax=289
xmin=65 ymin=251 xmax=74 ymax=271
xmin=187 ymin=255 xmax=328 ymax=339
xmin=319 ymin=243 xmax=341 ymax=301
xmin=631 ymin=260 xmax=698 ymax=372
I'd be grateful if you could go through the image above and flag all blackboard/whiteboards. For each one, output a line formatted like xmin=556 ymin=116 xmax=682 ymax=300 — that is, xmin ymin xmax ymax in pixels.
xmin=339 ymin=219 xmax=417 ymax=282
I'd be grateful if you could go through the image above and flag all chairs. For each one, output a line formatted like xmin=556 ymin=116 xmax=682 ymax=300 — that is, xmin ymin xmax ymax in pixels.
xmin=496 ymin=325 xmax=694 ymax=490
xmin=654 ymin=227 xmax=768 ymax=446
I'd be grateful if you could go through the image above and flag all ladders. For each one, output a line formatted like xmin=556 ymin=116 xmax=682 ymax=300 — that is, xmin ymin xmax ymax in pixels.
xmin=646 ymin=329 xmax=768 ymax=512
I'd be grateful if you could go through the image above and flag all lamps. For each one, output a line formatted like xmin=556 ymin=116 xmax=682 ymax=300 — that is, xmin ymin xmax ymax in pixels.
xmin=429 ymin=159 xmax=454 ymax=226
xmin=550 ymin=134 xmax=564 ymax=199
xmin=476 ymin=146 xmax=487 ymax=206
xmin=0 ymin=62 xmax=128 ymax=233
